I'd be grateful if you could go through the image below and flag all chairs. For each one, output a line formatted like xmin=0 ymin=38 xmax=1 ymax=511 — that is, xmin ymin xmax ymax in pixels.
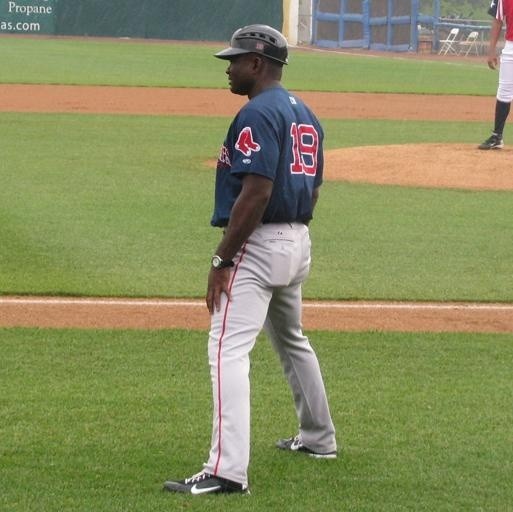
xmin=458 ymin=32 xmax=479 ymax=56
xmin=438 ymin=28 xmax=459 ymax=56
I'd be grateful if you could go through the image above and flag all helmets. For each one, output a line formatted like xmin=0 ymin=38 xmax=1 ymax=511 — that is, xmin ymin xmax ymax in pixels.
xmin=213 ymin=24 xmax=288 ymax=65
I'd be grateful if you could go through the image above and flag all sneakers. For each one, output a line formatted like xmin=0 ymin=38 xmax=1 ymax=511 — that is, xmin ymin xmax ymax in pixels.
xmin=478 ymin=130 xmax=503 ymax=150
xmin=274 ymin=435 xmax=336 ymax=459
xmin=163 ymin=471 xmax=247 ymax=494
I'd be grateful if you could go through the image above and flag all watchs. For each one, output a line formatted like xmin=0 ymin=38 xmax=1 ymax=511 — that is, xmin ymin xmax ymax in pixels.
xmin=210 ymin=255 xmax=234 ymax=270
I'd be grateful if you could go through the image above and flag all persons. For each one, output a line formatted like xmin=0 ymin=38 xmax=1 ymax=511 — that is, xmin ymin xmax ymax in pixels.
xmin=163 ymin=25 xmax=338 ymax=495
xmin=478 ymin=0 xmax=513 ymax=150
